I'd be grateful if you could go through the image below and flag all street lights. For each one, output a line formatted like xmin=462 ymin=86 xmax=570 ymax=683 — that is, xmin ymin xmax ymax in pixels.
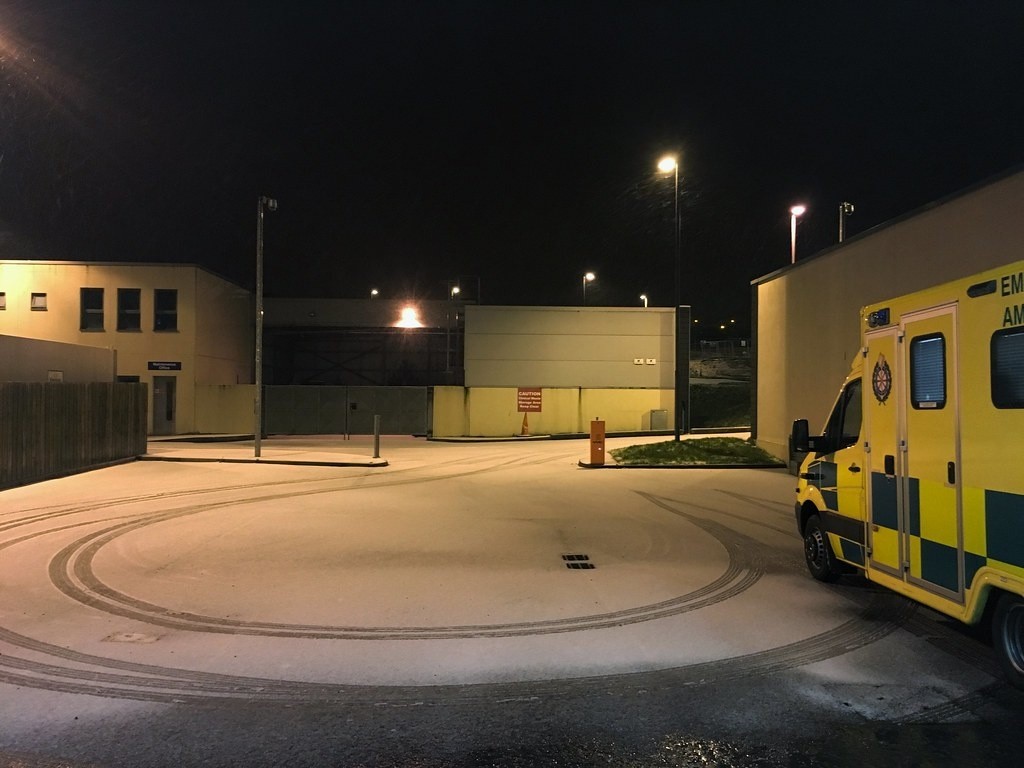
xmin=838 ymin=202 xmax=855 ymax=242
xmin=253 ymin=195 xmax=281 ymax=461
xmin=582 ymin=273 xmax=597 ymax=306
xmin=658 ymin=156 xmax=679 ymax=305
xmin=789 ymin=204 xmax=805 ymax=265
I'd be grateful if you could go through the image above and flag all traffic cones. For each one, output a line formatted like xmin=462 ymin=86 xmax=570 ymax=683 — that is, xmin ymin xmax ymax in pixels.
xmin=516 ymin=411 xmax=533 ymax=437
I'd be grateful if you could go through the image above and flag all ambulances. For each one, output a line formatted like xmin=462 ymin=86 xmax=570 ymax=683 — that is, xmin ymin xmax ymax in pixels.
xmin=788 ymin=260 xmax=1023 ymax=691
